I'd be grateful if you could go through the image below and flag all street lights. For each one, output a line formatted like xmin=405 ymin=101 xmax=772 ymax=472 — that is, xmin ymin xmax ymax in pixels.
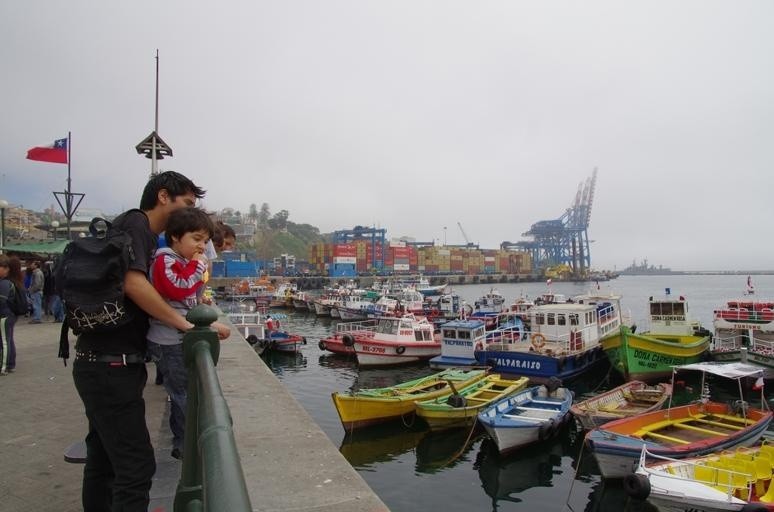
xmin=0 ymin=199 xmax=8 ymax=247
xmin=51 ymin=219 xmax=60 ymax=240
xmin=78 ymin=231 xmax=88 ymax=238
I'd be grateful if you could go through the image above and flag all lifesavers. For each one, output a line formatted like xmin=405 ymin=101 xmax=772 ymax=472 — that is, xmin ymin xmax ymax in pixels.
xmin=433 ymin=309 xmax=439 ymax=316
xmin=476 ymin=343 xmax=483 ymax=350
xmin=531 ymin=333 xmax=545 ymax=348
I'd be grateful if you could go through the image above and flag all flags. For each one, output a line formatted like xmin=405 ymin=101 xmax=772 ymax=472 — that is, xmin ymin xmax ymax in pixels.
xmin=25 ymin=138 xmax=67 ymax=164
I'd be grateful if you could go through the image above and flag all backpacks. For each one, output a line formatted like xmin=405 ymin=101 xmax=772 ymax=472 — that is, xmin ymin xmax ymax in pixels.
xmin=2 ymin=277 xmax=28 ymax=315
xmin=55 ymin=208 xmax=150 ymax=336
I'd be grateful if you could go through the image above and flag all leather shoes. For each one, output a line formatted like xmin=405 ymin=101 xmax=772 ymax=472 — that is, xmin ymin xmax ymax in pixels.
xmin=156 ymin=377 xmax=163 ymax=385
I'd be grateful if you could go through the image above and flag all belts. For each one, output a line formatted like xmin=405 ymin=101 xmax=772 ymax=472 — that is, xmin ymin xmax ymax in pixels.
xmin=75 ymin=351 xmax=146 ymax=363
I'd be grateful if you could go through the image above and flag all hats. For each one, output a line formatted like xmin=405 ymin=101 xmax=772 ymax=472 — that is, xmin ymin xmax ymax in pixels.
xmin=0 ymin=254 xmax=11 ymax=266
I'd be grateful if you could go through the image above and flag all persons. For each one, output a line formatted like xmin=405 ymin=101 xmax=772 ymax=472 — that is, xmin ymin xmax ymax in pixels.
xmin=1 ymin=251 xmax=64 ymax=376
xmin=72 ymin=171 xmax=236 ymax=512
xmin=263 ymin=314 xmax=274 ymax=340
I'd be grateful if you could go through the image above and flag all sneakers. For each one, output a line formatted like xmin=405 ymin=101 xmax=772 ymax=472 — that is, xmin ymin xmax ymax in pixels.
xmin=171 ymin=448 xmax=182 ymax=460
xmin=28 ymin=319 xmax=42 ymax=324
xmin=0 ymin=368 xmax=17 ymax=376
